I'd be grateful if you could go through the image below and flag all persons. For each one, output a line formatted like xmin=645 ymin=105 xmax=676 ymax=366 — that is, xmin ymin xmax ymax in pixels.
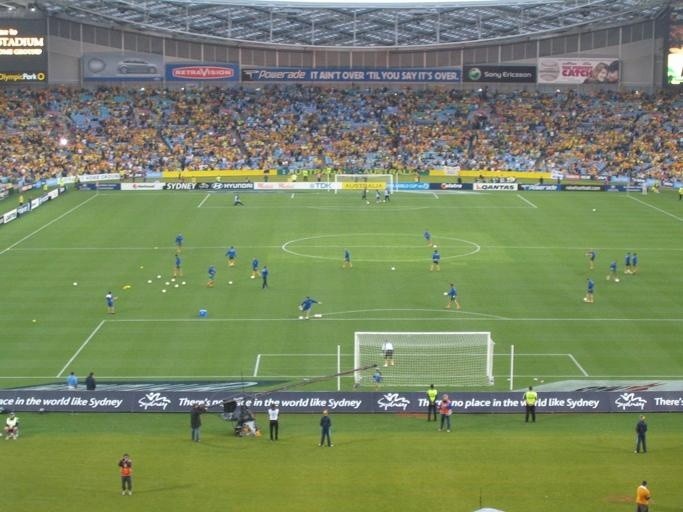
xmin=381 ymin=340 xmax=396 ymax=371
xmin=119 ymin=455 xmax=135 ymax=499
xmin=64 ymin=372 xmax=98 ymax=392
xmin=580 ymin=251 xmax=638 ymax=304
xmin=583 ymin=61 xmax=618 ymax=83
xmin=423 ymin=383 xmax=455 ymax=434
xmin=371 ymin=369 xmax=385 ymax=387
xmin=523 ymin=385 xmax=538 ymax=424
xmin=635 ymin=413 xmax=647 ymax=454
xmin=635 ymin=481 xmax=651 ymax=507
xmin=103 ymin=290 xmax=115 ymax=316
xmin=5 ymin=413 xmax=20 ymax=442
xmin=297 ymin=295 xmax=322 ymax=321
xmin=0 ymin=85 xmax=683 ymax=206
xmin=172 ymin=234 xmax=270 ymax=297
xmin=190 ymin=403 xmax=202 ymax=444
xmin=419 ymin=227 xmax=462 ymax=313
xmin=341 ymin=249 xmax=355 ymax=268
xmin=320 ymin=411 xmax=332 ymax=448
xmin=267 ymin=404 xmax=280 ymax=441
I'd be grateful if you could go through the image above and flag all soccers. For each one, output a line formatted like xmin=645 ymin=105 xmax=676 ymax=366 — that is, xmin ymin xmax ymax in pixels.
xmin=228 ymin=280 xmax=232 ymax=284
xmin=73 ymin=282 xmax=78 ymax=286
xmin=391 ymin=267 xmax=396 ymax=270
xmin=147 ymin=280 xmax=152 ymax=283
xmin=157 ymin=275 xmax=161 ymax=279
xmin=33 ymin=319 xmax=36 ymax=323
xmin=534 ymin=378 xmax=545 ymax=384
xmin=433 ymin=245 xmax=438 ymax=248
xmin=592 ymin=208 xmax=596 ymax=213
xmin=507 ymin=378 xmax=511 ymax=382
xmin=298 ymin=314 xmax=322 ymax=319
xmin=161 ymin=278 xmax=186 ymax=293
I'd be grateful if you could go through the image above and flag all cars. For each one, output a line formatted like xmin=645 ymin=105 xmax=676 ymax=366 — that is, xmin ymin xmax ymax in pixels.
xmin=118 ymin=58 xmax=157 ymax=75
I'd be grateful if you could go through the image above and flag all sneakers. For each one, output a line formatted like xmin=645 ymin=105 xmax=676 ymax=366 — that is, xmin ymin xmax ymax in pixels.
xmin=437 ymin=428 xmax=442 ymax=430
xmin=446 ymin=428 xmax=450 ymax=431
xmin=127 ymin=490 xmax=131 ymax=494
xmin=328 ymin=444 xmax=333 ymax=446
xmin=317 ymin=443 xmax=323 ymax=447
xmin=4 ymin=435 xmax=10 ymax=440
xmin=121 ymin=490 xmax=125 ymax=495
xmin=12 ymin=434 xmax=15 ymax=439
